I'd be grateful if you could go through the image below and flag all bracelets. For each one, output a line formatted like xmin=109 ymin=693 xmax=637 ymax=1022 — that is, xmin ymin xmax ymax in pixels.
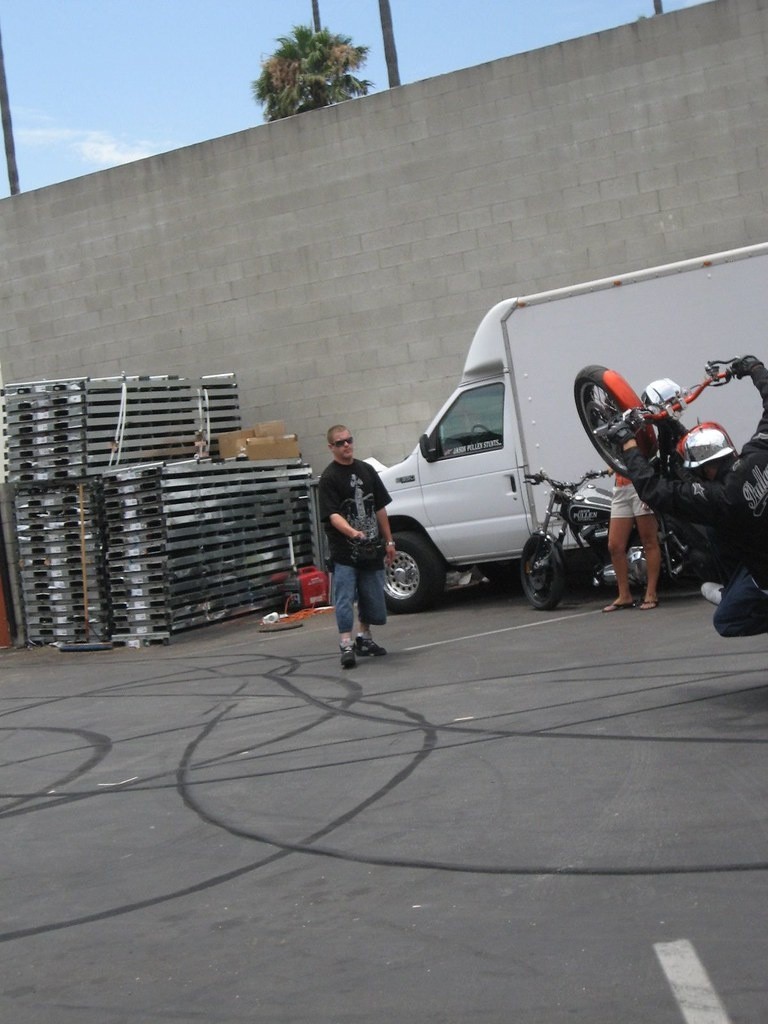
xmin=386 ymin=542 xmax=395 ymax=546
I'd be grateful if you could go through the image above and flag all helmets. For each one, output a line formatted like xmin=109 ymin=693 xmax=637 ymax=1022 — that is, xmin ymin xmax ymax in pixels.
xmin=683 ymin=427 xmax=733 ymax=468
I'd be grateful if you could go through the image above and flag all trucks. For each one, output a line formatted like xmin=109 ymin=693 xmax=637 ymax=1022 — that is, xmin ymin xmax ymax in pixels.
xmin=378 ymin=243 xmax=768 ymax=615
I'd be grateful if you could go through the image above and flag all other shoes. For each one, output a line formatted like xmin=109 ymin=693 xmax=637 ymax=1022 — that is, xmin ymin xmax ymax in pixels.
xmin=700 ymin=582 xmax=725 ymax=605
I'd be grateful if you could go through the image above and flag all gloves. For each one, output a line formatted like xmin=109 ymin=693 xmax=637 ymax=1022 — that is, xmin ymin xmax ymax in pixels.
xmin=607 ymin=414 xmax=636 ymax=447
xmin=731 ymin=354 xmax=764 ymax=380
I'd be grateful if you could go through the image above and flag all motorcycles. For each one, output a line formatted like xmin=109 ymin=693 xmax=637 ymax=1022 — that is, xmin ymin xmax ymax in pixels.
xmin=523 ymin=356 xmax=747 ymax=611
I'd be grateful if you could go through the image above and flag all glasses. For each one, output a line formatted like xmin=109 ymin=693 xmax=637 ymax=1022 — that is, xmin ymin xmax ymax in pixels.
xmin=331 ymin=437 xmax=354 ymax=447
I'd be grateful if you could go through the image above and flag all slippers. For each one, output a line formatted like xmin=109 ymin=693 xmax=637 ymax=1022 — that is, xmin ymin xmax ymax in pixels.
xmin=601 ymin=603 xmax=635 ymax=613
xmin=640 ymin=597 xmax=658 ymax=610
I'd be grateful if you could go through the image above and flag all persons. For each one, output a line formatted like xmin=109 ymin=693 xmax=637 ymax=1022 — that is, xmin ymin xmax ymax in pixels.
xmin=319 ymin=425 xmax=397 ymax=668
xmin=608 ymin=355 xmax=768 ymax=637
xmin=602 ymin=467 xmax=658 ymax=612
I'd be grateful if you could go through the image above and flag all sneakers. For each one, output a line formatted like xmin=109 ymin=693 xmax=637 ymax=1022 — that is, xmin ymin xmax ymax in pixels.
xmin=338 ymin=641 xmax=355 ymax=666
xmin=353 ymin=637 xmax=387 ymax=657
